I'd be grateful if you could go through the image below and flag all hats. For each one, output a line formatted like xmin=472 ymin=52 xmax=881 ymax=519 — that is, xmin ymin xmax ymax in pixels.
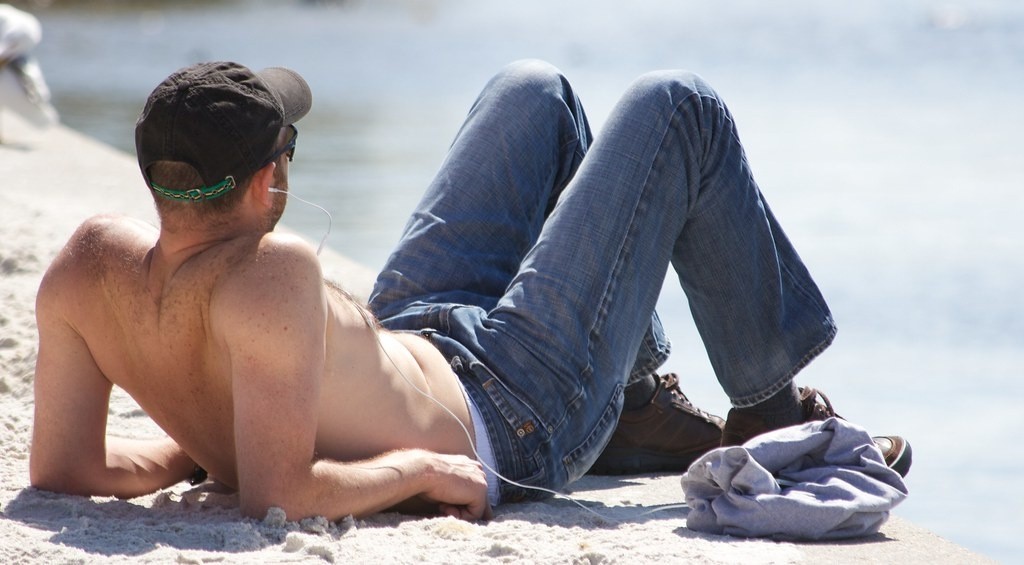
xmin=132 ymin=61 xmax=314 ymax=203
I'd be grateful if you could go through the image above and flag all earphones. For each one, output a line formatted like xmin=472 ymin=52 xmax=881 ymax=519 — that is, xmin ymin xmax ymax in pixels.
xmin=268 ymin=188 xmax=279 ymax=193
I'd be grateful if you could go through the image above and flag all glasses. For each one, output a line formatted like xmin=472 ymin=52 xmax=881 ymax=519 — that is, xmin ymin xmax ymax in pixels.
xmin=259 ymin=126 xmax=297 ymax=175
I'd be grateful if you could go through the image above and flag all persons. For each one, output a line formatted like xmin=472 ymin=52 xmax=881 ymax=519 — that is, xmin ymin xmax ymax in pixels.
xmin=29 ymin=58 xmax=912 ymax=522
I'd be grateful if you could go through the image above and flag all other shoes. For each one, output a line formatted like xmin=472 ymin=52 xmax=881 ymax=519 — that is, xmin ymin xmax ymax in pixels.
xmin=584 ymin=372 xmax=726 ymax=476
xmin=720 ymin=387 xmax=912 ymax=489
